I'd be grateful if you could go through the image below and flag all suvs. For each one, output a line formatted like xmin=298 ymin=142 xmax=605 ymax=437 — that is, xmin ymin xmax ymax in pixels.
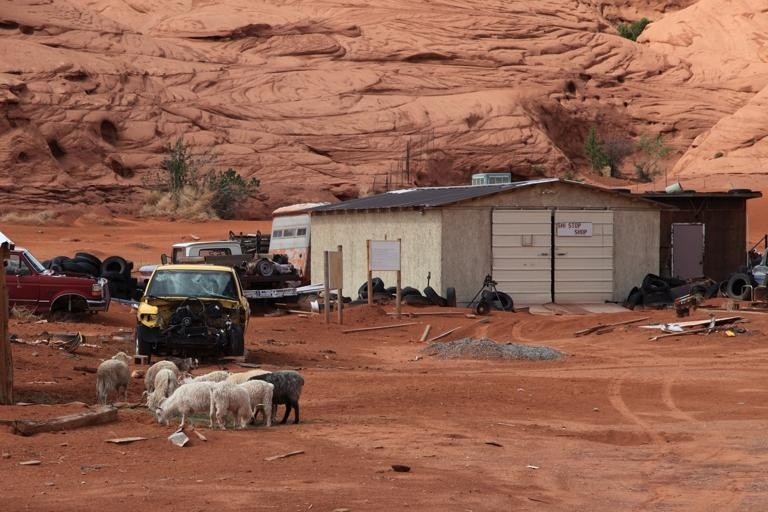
xmin=4 ymin=247 xmax=111 ymax=323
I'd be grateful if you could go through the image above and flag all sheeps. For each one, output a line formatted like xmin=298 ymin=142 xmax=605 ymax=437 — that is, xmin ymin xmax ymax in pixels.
xmin=142 ymin=357 xmax=306 ymax=432
xmin=95 ymin=351 xmax=134 ymax=403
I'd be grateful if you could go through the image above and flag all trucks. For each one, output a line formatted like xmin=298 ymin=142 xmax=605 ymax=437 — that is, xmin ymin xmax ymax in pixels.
xmin=137 ymin=240 xmax=299 ymax=312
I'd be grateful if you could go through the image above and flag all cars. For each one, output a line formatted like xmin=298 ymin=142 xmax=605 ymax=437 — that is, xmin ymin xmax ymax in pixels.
xmin=131 ymin=263 xmax=251 ymax=364
xmin=750 ymin=247 xmax=768 ymax=285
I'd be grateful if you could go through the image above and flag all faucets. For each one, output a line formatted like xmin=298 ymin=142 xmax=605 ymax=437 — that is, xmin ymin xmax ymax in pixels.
xmin=740 ymin=285 xmax=754 ymax=301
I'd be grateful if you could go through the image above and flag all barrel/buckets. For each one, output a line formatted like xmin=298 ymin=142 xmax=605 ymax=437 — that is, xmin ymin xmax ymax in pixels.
xmin=665 ymin=183 xmax=684 ymax=195
